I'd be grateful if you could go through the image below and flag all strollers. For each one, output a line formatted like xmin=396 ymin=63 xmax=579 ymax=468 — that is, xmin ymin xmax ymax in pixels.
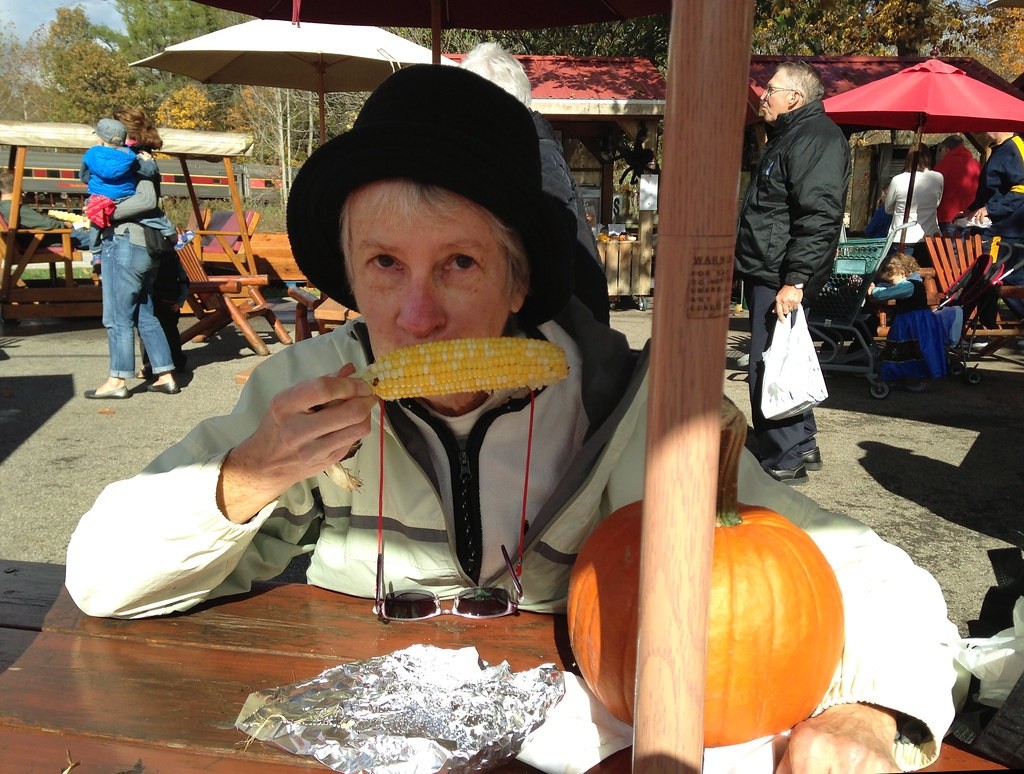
xmin=906 ymin=241 xmax=1024 ymax=392
xmin=805 ymin=219 xmax=918 ymax=400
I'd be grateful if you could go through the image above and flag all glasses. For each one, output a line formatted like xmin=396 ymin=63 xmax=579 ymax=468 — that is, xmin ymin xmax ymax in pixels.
xmin=372 ymin=543 xmax=524 ymax=623
xmin=766 ymin=85 xmax=804 ymax=99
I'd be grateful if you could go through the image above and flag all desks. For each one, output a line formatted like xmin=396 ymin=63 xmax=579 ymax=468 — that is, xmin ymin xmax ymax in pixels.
xmin=0 ymin=558 xmax=1012 ymax=774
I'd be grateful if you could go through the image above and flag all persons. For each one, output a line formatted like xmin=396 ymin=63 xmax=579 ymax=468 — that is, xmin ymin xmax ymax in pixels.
xmin=0 ymin=105 xmax=196 ymax=400
xmin=62 ymin=64 xmax=961 ymax=774
xmin=465 ymin=43 xmax=1024 ymax=487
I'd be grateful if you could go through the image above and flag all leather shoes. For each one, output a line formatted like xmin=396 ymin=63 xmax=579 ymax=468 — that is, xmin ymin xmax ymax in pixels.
xmin=764 ymin=446 xmax=821 ymax=486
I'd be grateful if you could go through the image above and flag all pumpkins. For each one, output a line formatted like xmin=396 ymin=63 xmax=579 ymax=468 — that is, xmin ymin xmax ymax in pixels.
xmin=567 ymin=412 xmax=846 ymax=749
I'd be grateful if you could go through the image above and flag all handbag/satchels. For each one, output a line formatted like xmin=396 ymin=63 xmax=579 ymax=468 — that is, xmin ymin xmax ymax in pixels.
xmin=951 ymin=593 xmax=1024 ymax=711
xmin=760 ymin=304 xmax=828 ymax=420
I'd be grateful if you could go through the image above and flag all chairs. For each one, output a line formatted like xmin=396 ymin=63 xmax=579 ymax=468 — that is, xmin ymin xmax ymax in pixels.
xmin=287 ymin=279 xmax=341 ymax=340
xmin=175 ymin=227 xmax=293 ymax=355
xmin=186 ymin=208 xmax=212 ymax=231
xmin=191 ymin=210 xmax=261 ymax=272
xmin=925 ymin=229 xmax=1024 ymax=356
xmin=0 ymin=212 xmax=82 ymax=305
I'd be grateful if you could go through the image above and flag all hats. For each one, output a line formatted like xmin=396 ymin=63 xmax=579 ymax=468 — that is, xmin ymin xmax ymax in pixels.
xmin=143 ymin=226 xmax=174 ymax=257
xmin=286 ymin=63 xmax=578 ymax=331
xmin=92 ymin=118 xmax=126 ymax=146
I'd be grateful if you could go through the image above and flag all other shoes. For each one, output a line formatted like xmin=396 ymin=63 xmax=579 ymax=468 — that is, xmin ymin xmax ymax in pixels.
xmin=84 ymin=385 xmax=129 ymax=399
xmin=91 ymin=253 xmax=101 ymax=269
xmin=173 ymin=229 xmax=194 ymax=251
xmin=145 ymin=380 xmax=180 ymax=394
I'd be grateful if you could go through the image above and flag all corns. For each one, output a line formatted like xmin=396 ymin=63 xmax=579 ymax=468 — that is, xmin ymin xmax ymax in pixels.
xmin=368 ymin=336 xmax=568 ymax=400
xmin=48 ymin=210 xmax=83 ymax=222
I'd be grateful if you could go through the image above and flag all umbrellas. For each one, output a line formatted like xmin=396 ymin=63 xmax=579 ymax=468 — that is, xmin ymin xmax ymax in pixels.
xmin=127 ymin=19 xmax=464 ymax=147
xmin=824 ymin=59 xmax=1024 ymax=255
